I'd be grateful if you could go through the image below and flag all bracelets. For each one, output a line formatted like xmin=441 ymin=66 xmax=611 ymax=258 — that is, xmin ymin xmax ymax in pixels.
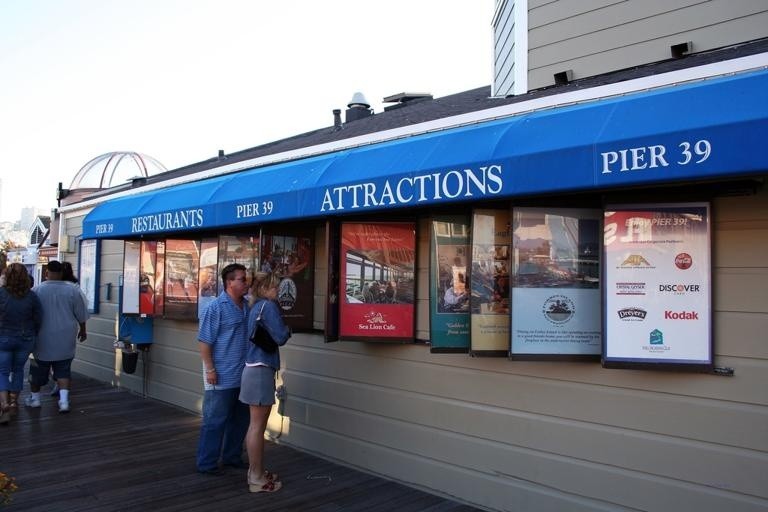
xmin=205 ymin=368 xmax=216 ymax=374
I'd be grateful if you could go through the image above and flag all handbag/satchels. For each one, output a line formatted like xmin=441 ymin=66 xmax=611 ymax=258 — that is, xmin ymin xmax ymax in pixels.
xmin=249 ymin=299 xmax=279 ymax=354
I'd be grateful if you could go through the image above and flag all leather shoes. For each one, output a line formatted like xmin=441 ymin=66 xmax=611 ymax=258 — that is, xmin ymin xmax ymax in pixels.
xmin=223 ymin=456 xmax=249 ymax=469
xmin=196 ymin=462 xmax=225 ymax=476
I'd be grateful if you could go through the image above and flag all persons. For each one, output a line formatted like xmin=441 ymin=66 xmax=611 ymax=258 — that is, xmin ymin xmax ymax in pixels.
xmin=262 ymin=238 xmax=313 ymax=280
xmin=238 ymin=270 xmax=292 ymax=495
xmin=191 ymin=262 xmax=250 ymax=476
xmin=358 ymin=275 xmax=395 ymax=304
xmin=440 ymin=244 xmax=510 ymax=315
xmin=0 ymin=249 xmax=90 ymax=425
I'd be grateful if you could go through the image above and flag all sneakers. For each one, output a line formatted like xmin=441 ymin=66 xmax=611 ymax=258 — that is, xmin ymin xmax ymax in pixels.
xmin=24 ymin=396 xmax=40 ymax=409
xmin=58 ymin=399 xmax=70 ymax=411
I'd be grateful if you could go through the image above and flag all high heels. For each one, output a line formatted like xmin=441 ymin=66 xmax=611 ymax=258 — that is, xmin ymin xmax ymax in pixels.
xmin=247 ymin=469 xmax=282 ymax=492
xmin=0 ymin=403 xmax=19 ymax=423
